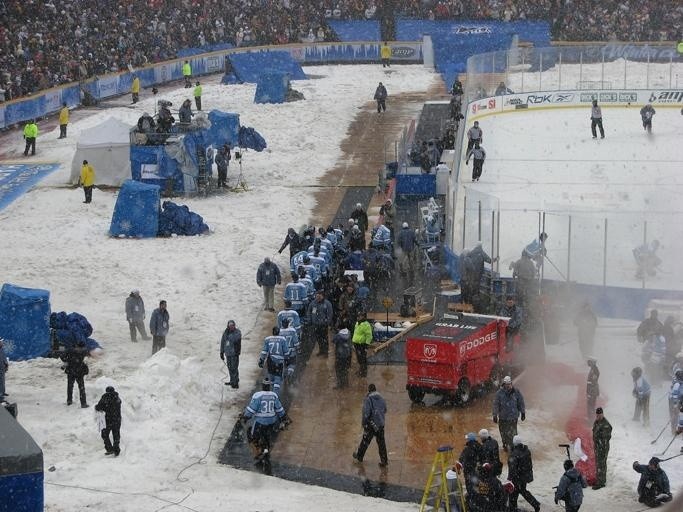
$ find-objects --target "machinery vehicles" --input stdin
[401,311,519,408]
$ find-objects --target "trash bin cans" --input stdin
[436,166,452,194]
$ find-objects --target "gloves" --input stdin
[521,411,525,421]
[492,415,497,423]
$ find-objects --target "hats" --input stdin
[368,384,376,392]
[464,432,475,440]
[649,458,659,469]
[348,198,408,230]
[503,375,512,384]
[478,428,489,438]
[512,434,521,445]
[596,407,603,414]
[563,460,574,470]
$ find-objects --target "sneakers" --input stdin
[353,451,362,461]
[379,462,388,467]
[252,452,265,465]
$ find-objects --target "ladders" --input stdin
[420,446,467,512]
